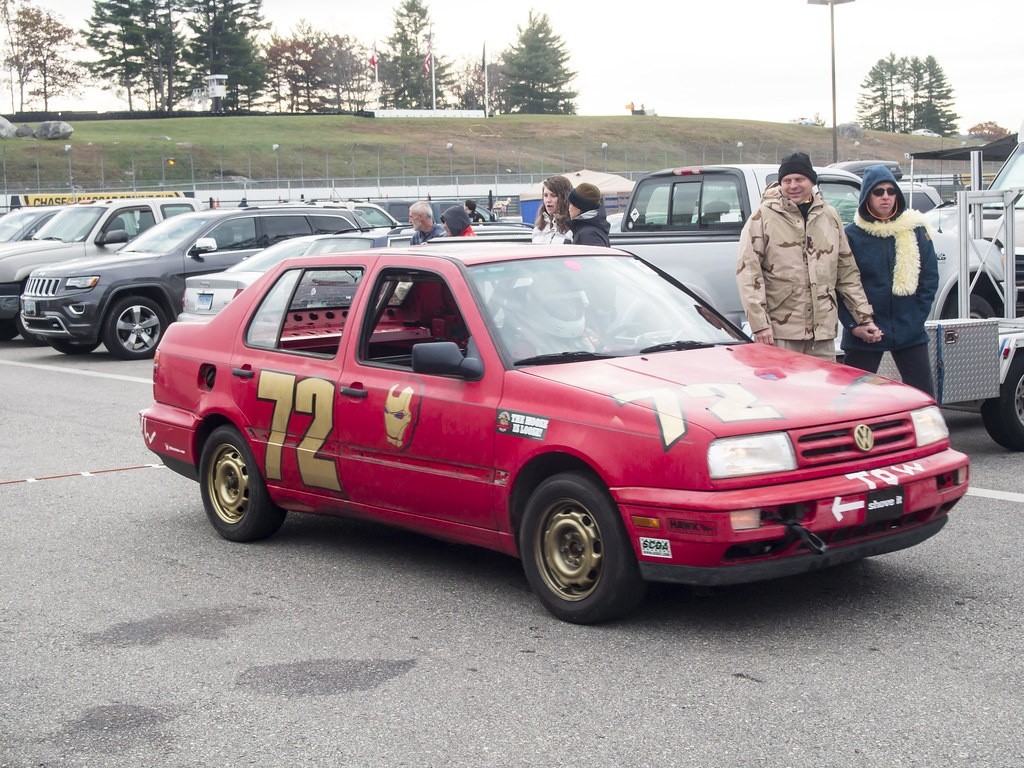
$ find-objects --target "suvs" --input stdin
[19,198,370,362]
[278,198,404,241]
[362,197,501,233]
[302,221,542,301]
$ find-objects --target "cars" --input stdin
[911,128,943,138]
[170,231,332,346]
[137,241,970,628]
[0,206,138,246]
[0,197,198,346]
[604,211,667,238]
[823,139,1024,318]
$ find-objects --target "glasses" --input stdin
[870,187,898,196]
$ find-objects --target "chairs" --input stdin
[696,199,731,223]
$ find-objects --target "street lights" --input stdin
[804,0,859,161]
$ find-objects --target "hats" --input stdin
[568,182,601,212]
[777,152,818,186]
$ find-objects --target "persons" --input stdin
[735,151,881,363]
[565,182,617,337]
[238,198,248,207]
[408,201,448,246]
[463,200,486,222]
[833,164,940,400]
[531,175,573,245]
[440,205,478,238]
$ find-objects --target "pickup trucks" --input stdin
[596,161,1009,371]
